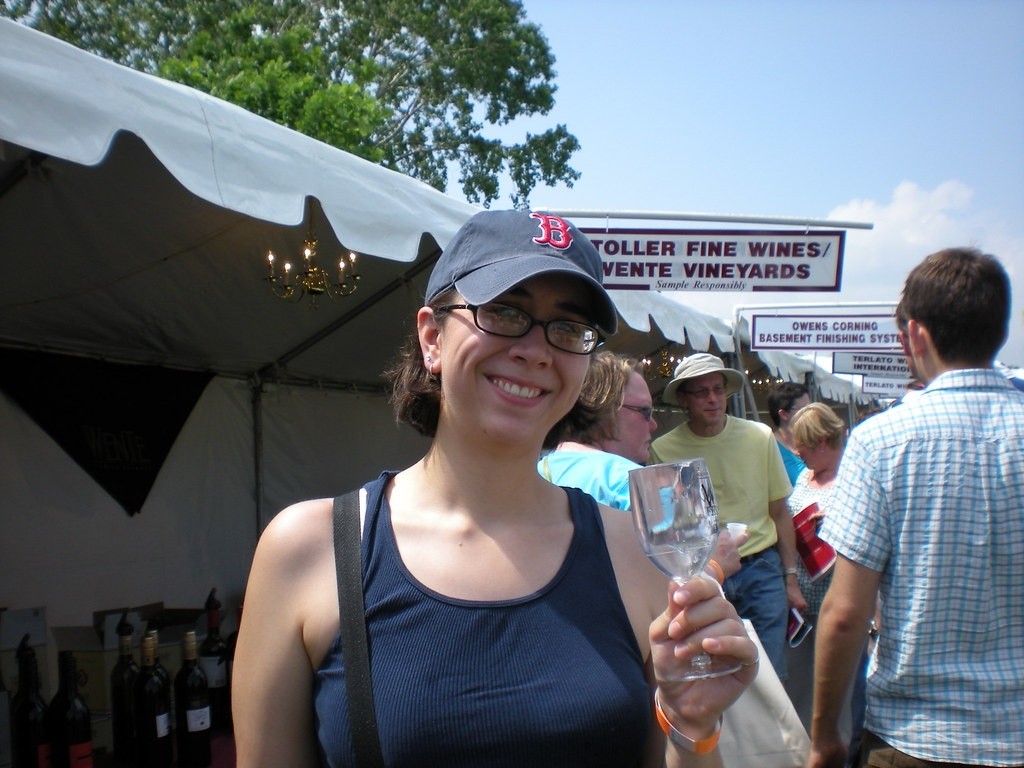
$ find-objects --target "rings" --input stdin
[740,655,759,670]
[818,513,821,518]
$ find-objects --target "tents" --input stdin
[0,14,882,626]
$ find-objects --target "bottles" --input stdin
[109,622,142,768]
[9,648,46,767]
[130,634,171,768]
[43,649,94,768]
[226,606,243,736]
[173,631,212,768]
[145,630,171,709]
[198,600,227,734]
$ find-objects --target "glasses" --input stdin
[676,383,727,399]
[435,302,608,355]
[618,403,652,422]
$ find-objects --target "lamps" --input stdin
[742,368,784,396]
[635,347,688,383]
[266,195,363,310]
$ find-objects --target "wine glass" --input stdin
[627,457,743,682]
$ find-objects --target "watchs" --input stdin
[869,619,879,643]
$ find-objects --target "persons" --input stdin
[231,211,757,768]
[538,353,870,685]
[806,250,1024,768]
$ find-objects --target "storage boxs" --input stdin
[0,601,238,768]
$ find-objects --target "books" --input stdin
[786,608,812,648]
[792,501,838,585]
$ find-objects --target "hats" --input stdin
[663,352,744,407]
[426,209,618,337]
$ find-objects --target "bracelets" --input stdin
[654,686,724,754]
[707,558,725,587]
[784,566,802,577]
